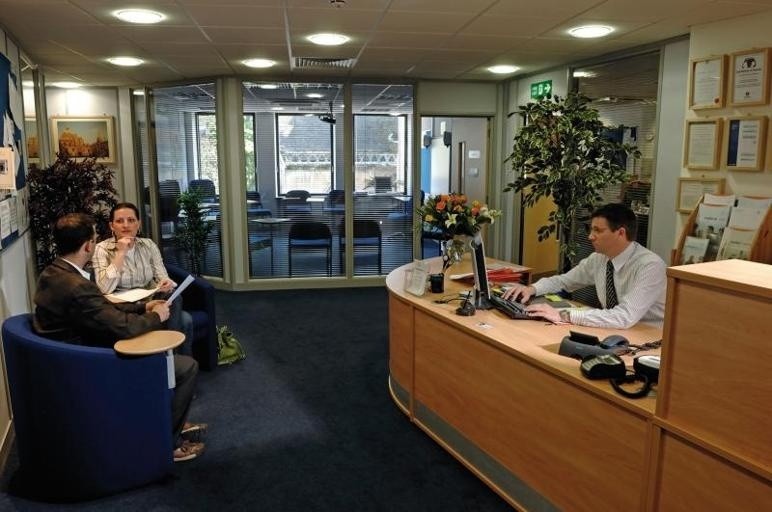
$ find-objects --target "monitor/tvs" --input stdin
[460,234,490,311]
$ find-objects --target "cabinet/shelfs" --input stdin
[670,192,772,266]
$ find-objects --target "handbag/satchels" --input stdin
[217,332,246,366]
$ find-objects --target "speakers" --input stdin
[443,131,452,146]
[423,133,430,146]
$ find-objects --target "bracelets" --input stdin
[559,309,570,324]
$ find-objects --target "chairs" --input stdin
[388,187,445,260]
[320,189,354,229]
[145,178,220,276]
[3,313,175,501]
[246,190,273,239]
[215,215,276,277]
[283,190,313,221]
[338,219,383,275]
[286,221,332,276]
[85,263,217,372]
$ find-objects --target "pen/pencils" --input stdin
[463,291,470,309]
[110,242,118,243]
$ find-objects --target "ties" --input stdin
[605,259,618,309]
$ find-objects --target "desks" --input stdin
[384,252,772,512]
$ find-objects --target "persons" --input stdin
[719,227,724,237]
[91,203,203,401]
[691,223,698,236]
[539,85,542,92]
[706,226,718,235]
[685,255,696,265]
[31,213,207,462]
[502,202,667,331]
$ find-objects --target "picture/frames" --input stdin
[50,116,116,165]
[688,55,727,110]
[721,116,767,172]
[727,48,770,106]
[683,117,723,170]
[25,117,42,164]
[675,176,724,214]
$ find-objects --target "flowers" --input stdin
[412,193,504,262]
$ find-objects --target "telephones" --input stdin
[558,330,634,361]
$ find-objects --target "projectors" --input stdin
[320,115,337,124]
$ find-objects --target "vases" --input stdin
[442,239,464,271]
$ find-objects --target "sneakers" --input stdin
[173,439,206,462]
[180,422,208,441]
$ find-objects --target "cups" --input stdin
[427,273,443,293]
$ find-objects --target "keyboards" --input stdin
[491,292,540,320]
